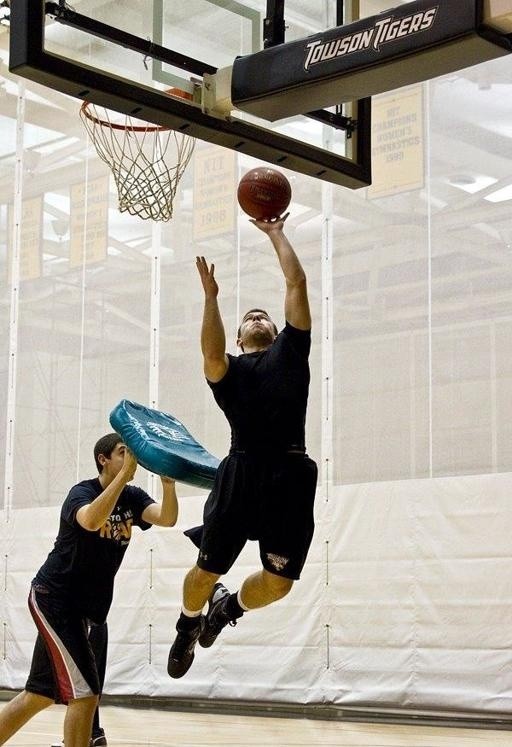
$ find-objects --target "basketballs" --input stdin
[237,167,291,220]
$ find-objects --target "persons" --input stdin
[0,430,179,747]
[51,621,108,747]
[163,211,319,680]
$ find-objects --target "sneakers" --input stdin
[167,610,207,677]
[198,578,230,644]
[51,726,108,747]
